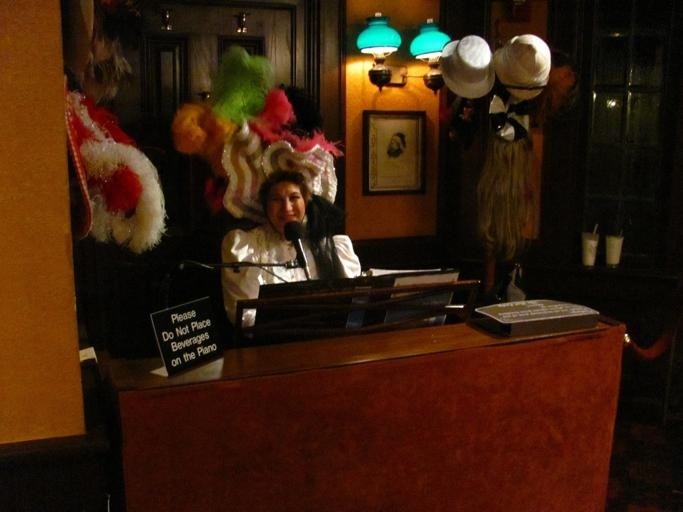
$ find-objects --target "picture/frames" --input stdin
[362,109,427,196]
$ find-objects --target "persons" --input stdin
[218,167,368,347]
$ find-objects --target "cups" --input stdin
[605,235,624,267]
[579,232,600,267]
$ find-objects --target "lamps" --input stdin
[235,10,248,34]
[356,16,407,92]
[159,7,174,32]
[410,21,451,96]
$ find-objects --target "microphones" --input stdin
[284,220,312,281]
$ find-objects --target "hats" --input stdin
[495,34,553,100]
[437,35,495,101]
